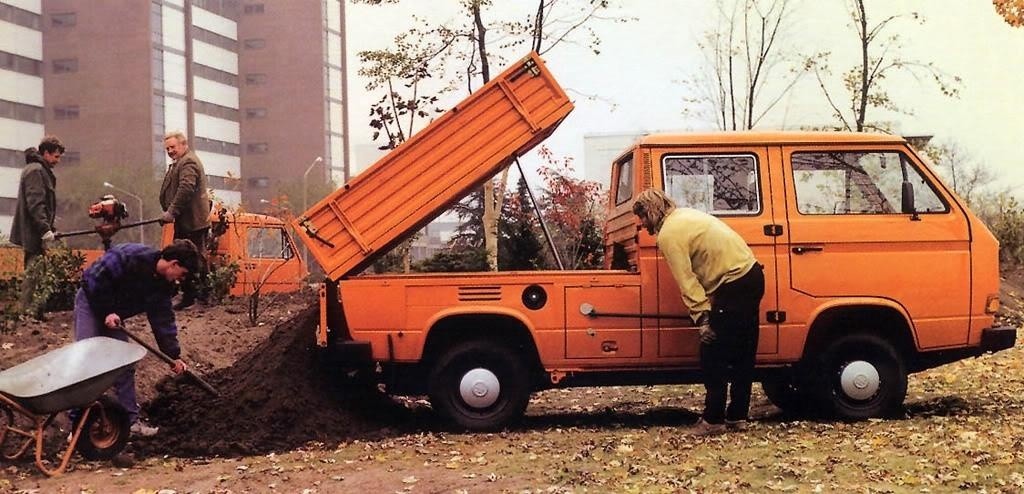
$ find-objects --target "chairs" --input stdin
[748,182,759,213]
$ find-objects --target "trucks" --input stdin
[0,207,310,303]
[293,54,1018,433]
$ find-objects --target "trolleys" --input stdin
[0,337,150,480]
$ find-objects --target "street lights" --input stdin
[302,156,322,274]
[260,197,277,208]
[104,180,144,245]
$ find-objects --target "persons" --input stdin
[9,134,66,322]
[65,238,200,442]
[158,131,211,310]
[632,187,765,434]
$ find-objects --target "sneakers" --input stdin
[129,418,160,440]
[682,416,749,436]
